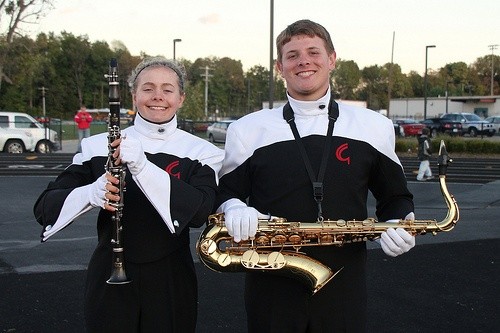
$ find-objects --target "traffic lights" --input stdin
[38,87,49,99]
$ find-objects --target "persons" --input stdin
[74,105,93,154]
[416,128,435,181]
[213,20,415,333]
[32,58,225,333]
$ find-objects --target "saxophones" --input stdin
[194,137,461,295]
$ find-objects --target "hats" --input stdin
[422,127,430,134]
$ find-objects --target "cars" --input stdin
[396,119,426,137]
[485,117,500,135]
[420,118,463,138]
[0,127,33,153]
[207,120,233,143]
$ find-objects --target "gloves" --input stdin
[225,204,276,242]
[380,211,416,256]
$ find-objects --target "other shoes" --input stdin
[418,177,426,181]
[427,175,434,179]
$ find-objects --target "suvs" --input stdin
[439,112,494,137]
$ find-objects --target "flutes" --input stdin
[103,56,135,286]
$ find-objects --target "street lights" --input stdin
[424,45,436,119]
[174,39,182,60]
[446,81,459,112]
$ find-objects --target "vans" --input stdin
[0,112,59,154]
